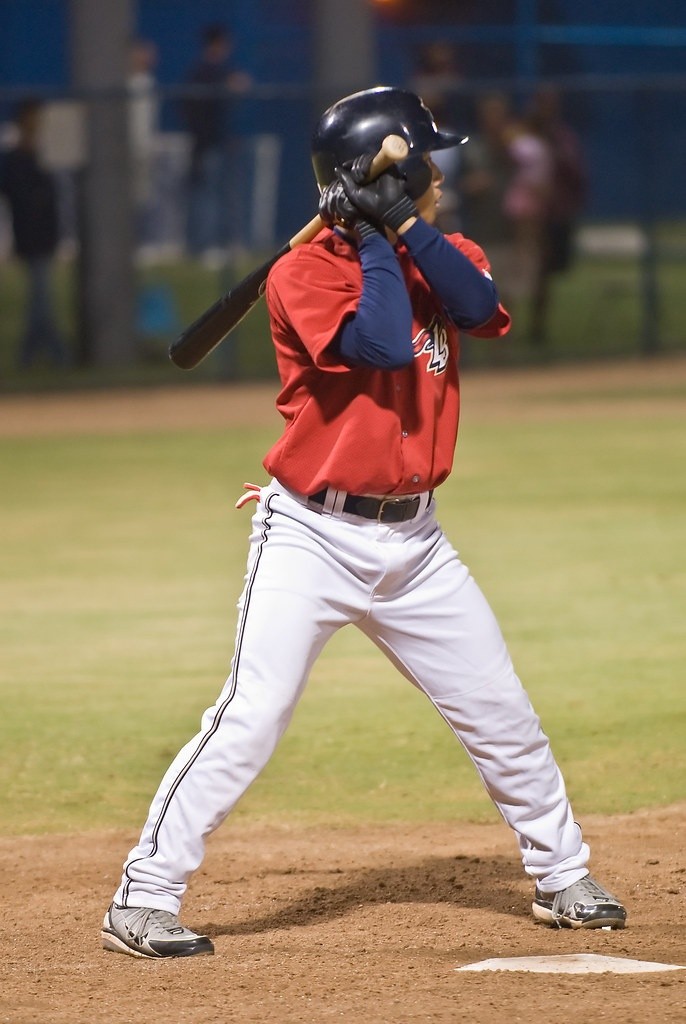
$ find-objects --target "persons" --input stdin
[96,85,626,958]
[0,22,592,374]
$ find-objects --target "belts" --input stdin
[308,486,434,523]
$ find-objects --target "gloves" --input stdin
[334,154,417,232]
[319,180,384,244]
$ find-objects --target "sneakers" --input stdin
[533,877,627,929]
[101,901,216,959]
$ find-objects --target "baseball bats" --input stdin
[167,134,408,373]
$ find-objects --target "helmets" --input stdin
[311,86,467,195]
